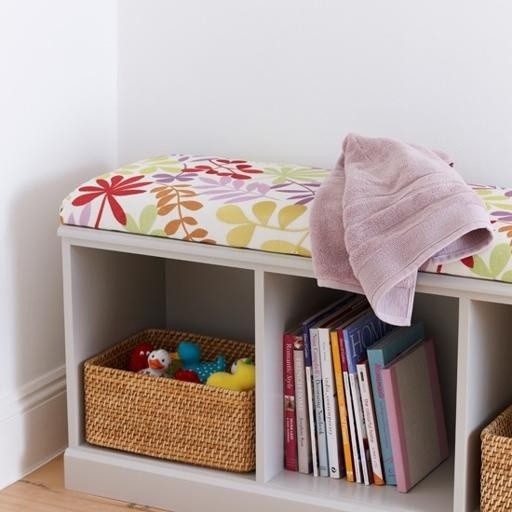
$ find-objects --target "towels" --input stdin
[308,135,494,329]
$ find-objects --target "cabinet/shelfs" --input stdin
[56,225,511,512]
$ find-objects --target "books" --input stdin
[283,293,450,493]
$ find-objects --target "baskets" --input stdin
[82,328,256,473]
[480,405,512,512]
